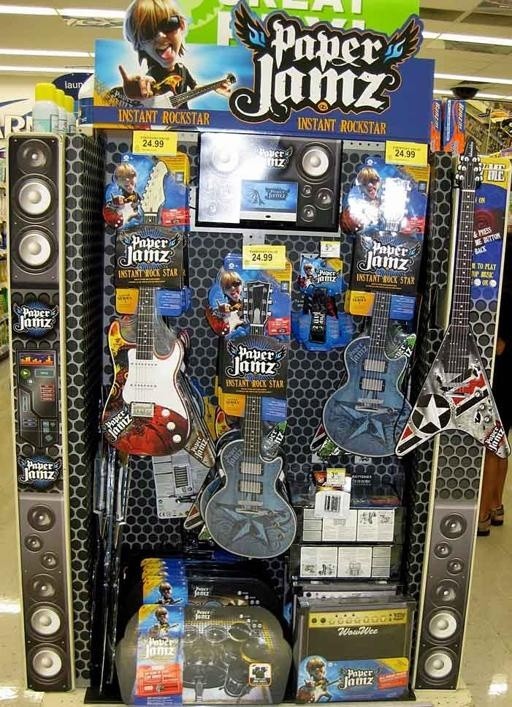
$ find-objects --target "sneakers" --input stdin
[478,506,504,536]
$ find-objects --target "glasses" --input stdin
[225,281,243,288]
[119,172,135,181]
[311,664,324,670]
[139,16,181,39]
[364,176,378,185]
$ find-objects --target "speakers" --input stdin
[199,132,340,231]
[19,494,72,695]
[10,133,60,285]
[411,504,480,690]
